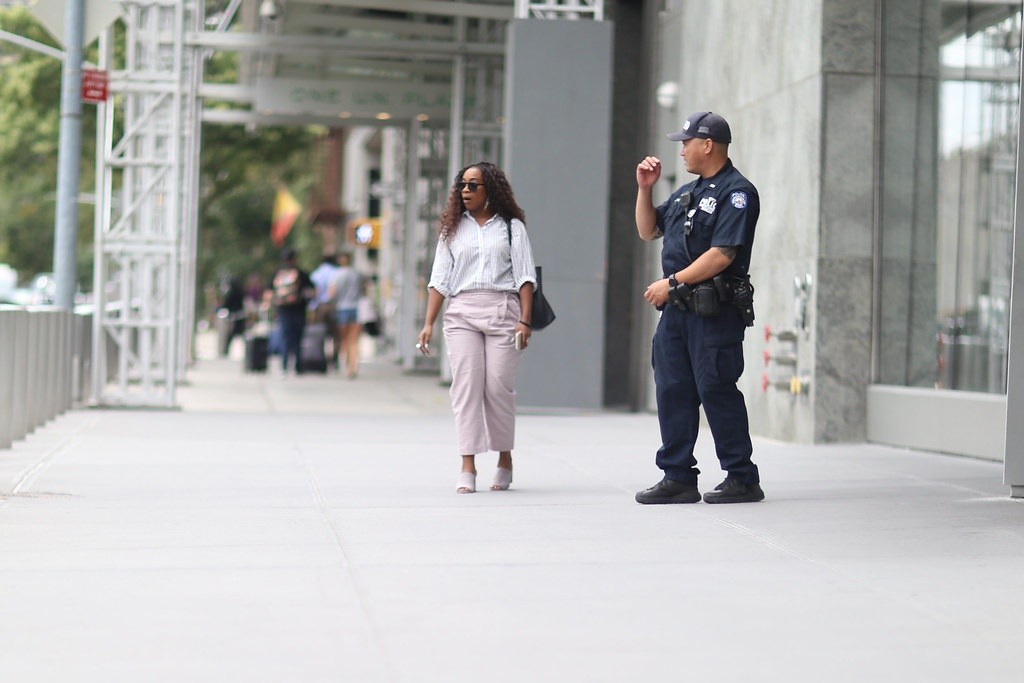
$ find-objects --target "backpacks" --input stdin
[272,268,300,306]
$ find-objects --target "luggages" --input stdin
[296,317,326,373]
[245,337,268,371]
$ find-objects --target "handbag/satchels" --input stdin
[508,218,556,330]
[355,298,378,324]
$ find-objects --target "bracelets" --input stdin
[518,320,531,328]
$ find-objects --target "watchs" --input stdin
[668,274,678,287]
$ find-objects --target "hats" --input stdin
[667,112,731,143]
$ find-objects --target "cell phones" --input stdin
[515,330,523,350]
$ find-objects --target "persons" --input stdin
[632,107,765,506]
[269,250,315,372]
[415,157,540,496]
[309,243,363,378]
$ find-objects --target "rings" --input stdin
[416,344,421,348]
[526,339,529,343]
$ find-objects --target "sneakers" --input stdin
[703,475,764,504]
[636,475,702,504]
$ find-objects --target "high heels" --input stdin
[456,468,476,493]
[489,464,512,490]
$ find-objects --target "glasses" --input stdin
[456,181,486,192]
